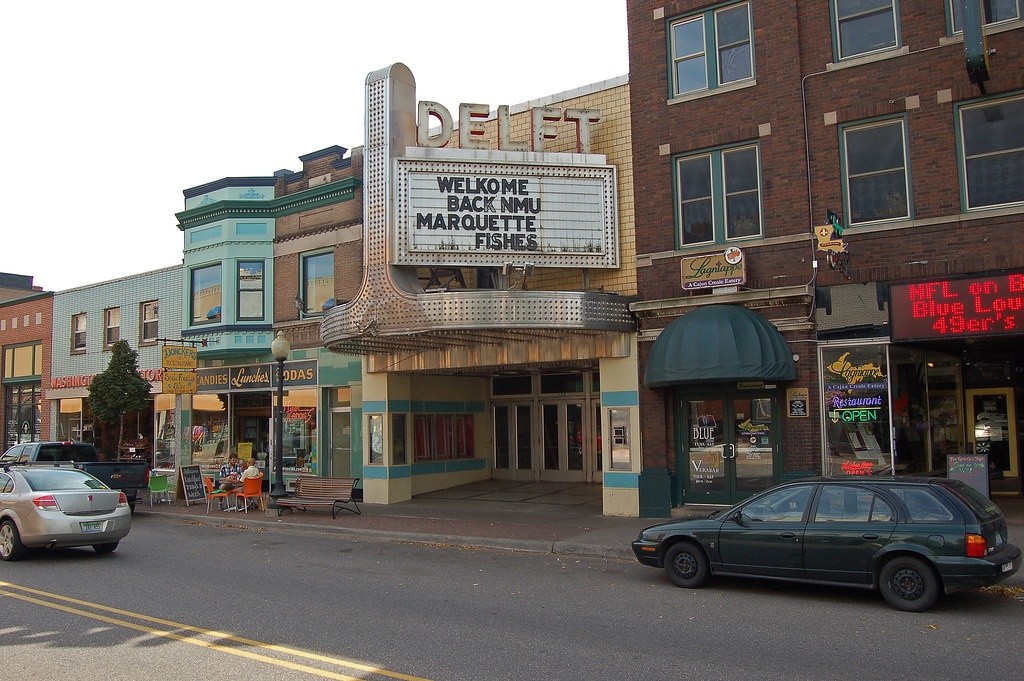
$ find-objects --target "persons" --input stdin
[227,458,259,509]
[218,454,248,511]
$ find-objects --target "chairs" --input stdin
[235,471,265,514]
[203,476,234,515]
[149,474,176,508]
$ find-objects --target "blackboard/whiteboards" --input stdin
[945,454,991,500]
[180,464,207,502]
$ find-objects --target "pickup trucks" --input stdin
[0,441,149,512]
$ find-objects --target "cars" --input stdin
[266,436,312,466]
[827,419,891,467]
[689,429,772,481]
[0,464,132,562]
[577,424,601,456]
[630,473,1023,613]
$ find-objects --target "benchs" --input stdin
[275,477,361,520]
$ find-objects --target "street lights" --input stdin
[268,330,290,509]
